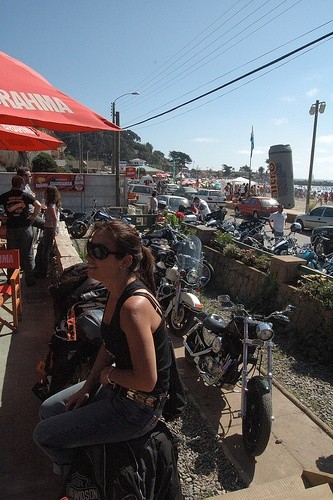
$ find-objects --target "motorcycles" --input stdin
[69,192,132,239]
[132,224,296,457]
[194,206,333,277]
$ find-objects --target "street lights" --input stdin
[110,92,140,174]
[305,100,327,212]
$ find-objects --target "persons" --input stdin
[147,190,158,214]
[223,182,260,196]
[33,220,170,469]
[195,180,202,190]
[157,181,168,195]
[269,204,286,242]
[0,176,42,286]
[16,166,44,270]
[32,186,62,277]
[191,196,211,215]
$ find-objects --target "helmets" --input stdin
[77,310,105,349]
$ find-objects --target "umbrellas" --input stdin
[0,124,64,151]
[0,51,123,132]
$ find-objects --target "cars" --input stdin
[234,196,284,220]
[310,226,333,256]
[127,182,227,223]
[294,205,333,230]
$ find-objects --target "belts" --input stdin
[120,389,154,407]
[122,388,168,408]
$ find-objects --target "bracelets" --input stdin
[107,367,120,385]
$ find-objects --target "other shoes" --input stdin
[34,271,47,278]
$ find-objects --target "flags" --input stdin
[250,126,254,158]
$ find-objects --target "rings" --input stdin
[66,403,72,407]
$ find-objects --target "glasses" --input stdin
[87,241,118,260]
[28,175,32,178]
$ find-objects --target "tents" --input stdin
[141,173,171,184]
[226,177,257,193]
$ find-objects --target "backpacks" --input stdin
[32,263,109,402]
[100,419,184,500]
